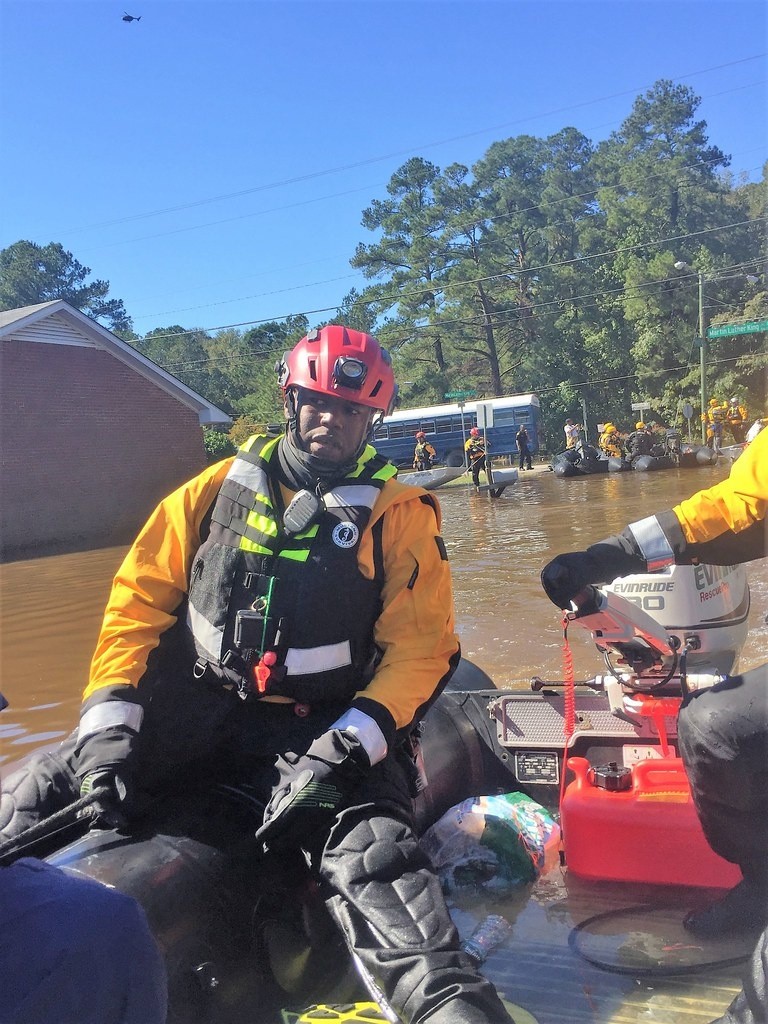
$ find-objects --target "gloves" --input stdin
[540,509,687,612]
[252,696,398,847]
[73,686,146,831]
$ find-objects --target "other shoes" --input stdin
[520,468,525,470]
[528,467,535,470]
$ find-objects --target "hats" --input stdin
[710,399,719,405]
[636,422,645,430]
[607,426,617,434]
[604,423,613,431]
[729,398,739,404]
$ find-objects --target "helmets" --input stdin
[415,431,426,439]
[470,427,480,438]
[274,323,401,416]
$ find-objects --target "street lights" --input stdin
[578,397,588,442]
[674,261,708,446]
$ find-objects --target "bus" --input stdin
[369,394,539,468]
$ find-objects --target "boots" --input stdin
[713,436,725,456]
[680,791,768,937]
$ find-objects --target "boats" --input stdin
[550,439,750,477]
[397,467,467,490]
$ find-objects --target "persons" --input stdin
[541,426,768,1024]
[465,428,491,487]
[0,323,513,1024]
[413,432,436,470]
[601,422,626,459]
[563,419,583,449]
[626,422,660,461]
[516,425,534,470]
[701,398,747,450]
